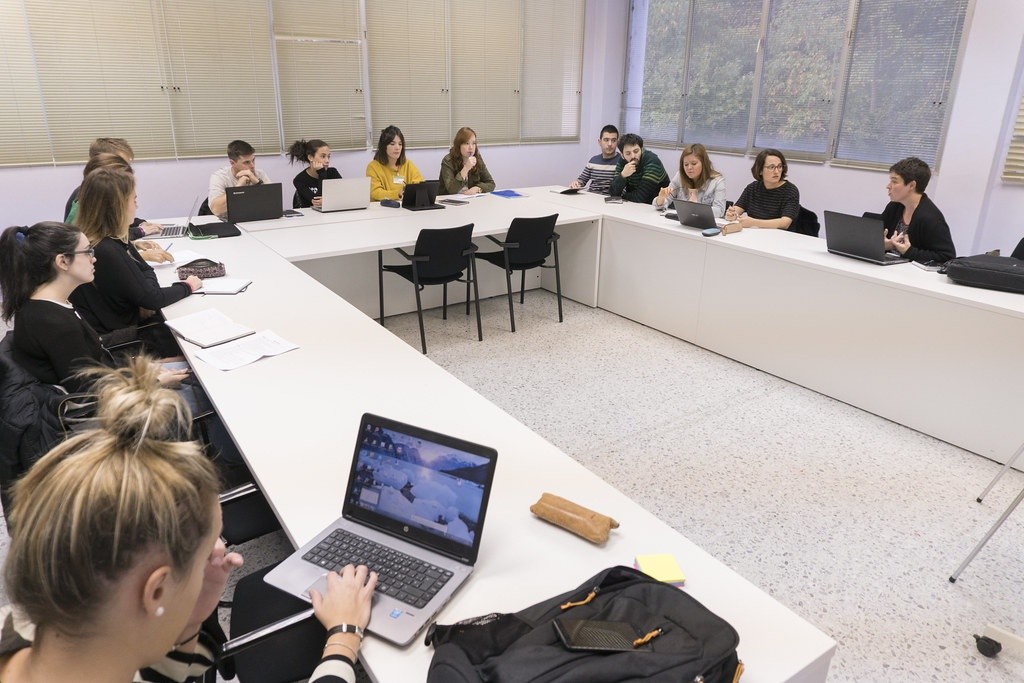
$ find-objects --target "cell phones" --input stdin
[552,618,653,653]
[161,361,193,373]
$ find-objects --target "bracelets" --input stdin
[322,642,358,662]
[478,187,482,193]
[325,623,363,642]
[174,633,199,649]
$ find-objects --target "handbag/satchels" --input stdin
[937,254,1024,293]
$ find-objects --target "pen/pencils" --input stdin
[165,243,172,251]
[470,152,471,156]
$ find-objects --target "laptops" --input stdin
[218,183,283,224]
[142,197,198,240]
[263,414,498,648]
[824,211,910,265]
[310,177,371,213]
[672,199,727,229]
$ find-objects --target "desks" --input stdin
[134,186,1024,682]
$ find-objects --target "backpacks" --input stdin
[424,565,744,683]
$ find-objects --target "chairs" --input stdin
[379,223,482,354]
[293,190,304,209]
[797,205,820,238]
[1,322,329,683]
[198,196,214,217]
[466,213,564,332]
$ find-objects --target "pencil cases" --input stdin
[720,223,742,236]
[177,258,226,280]
[530,492,620,543]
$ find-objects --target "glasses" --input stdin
[763,163,785,172]
[55,247,94,258]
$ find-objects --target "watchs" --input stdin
[255,178,263,185]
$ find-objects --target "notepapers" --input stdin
[634,554,686,588]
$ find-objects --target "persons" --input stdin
[208,140,272,218]
[437,127,496,196]
[64,137,202,356]
[653,144,727,219]
[725,148,800,231]
[0,222,246,474]
[366,125,425,202]
[570,125,626,196]
[880,158,956,265]
[609,133,674,210]
[285,139,343,208]
[0,351,378,683]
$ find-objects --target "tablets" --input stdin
[402,182,439,208]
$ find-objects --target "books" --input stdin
[660,209,677,216]
[913,259,941,271]
[164,307,255,349]
[439,199,469,206]
[282,209,303,217]
[604,197,623,204]
[551,187,585,194]
[191,277,253,295]
